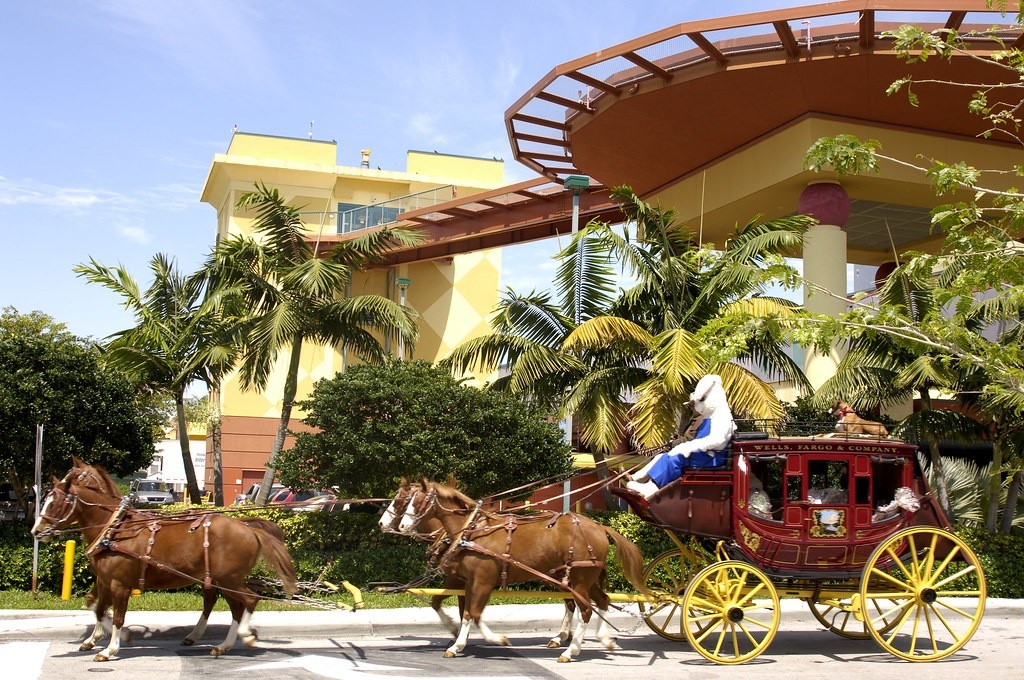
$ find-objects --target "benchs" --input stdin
[682,443,733,531]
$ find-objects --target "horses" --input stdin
[30,451,297,662]
[377,472,655,664]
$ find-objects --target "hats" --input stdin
[682,391,697,405]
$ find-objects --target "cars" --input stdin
[234,480,364,514]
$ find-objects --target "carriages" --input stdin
[31,417,988,666]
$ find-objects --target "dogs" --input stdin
[827,399,888,439]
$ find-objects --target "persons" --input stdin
[619,374,738,501]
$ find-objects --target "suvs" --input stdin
[128,476,175,510]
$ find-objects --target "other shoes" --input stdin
[619,466,633,483]
[626,479,660,501]
